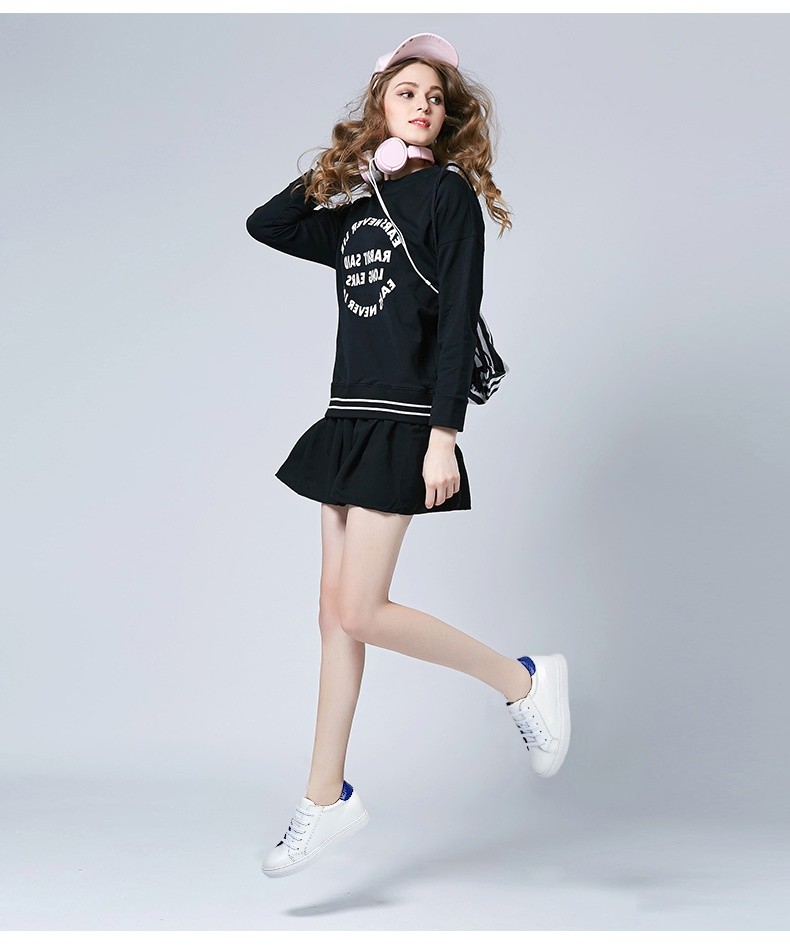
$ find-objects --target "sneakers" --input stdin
[262,782,369,879]
[372,33,458,90]
[507,653,572,778]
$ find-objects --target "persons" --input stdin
[239,54,578,880]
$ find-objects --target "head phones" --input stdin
[357,137,434,184]
